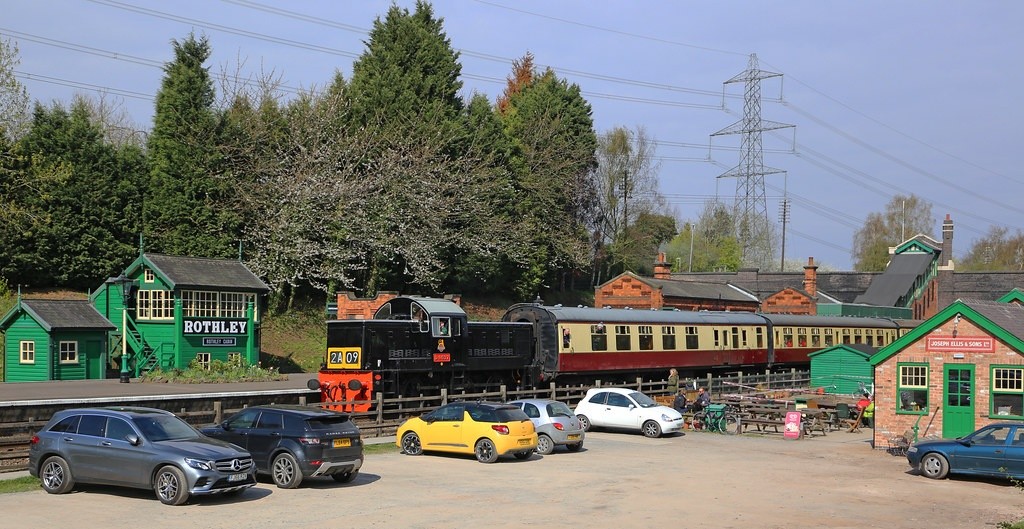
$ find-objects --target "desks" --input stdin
[743,407,828,438]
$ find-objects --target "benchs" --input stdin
[679,397,841,438]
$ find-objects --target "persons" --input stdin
[563,328,570,345]
[593,321,604,349]
[783,336,821,348]
[439,319,448,336]
[667,368,679,396]
[673,388,689,411]
[690,386,711,411]
[849,393,875,428]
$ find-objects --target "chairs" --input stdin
[984,435,996,444]
[448,409,459,421]
[844,406,866,434]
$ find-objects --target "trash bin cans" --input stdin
[704,403,727,431]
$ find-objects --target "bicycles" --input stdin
[691,398,739,435]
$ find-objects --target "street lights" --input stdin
[114,269,135,383]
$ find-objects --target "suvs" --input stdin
[197,403,364,489]
[28,406,258,506]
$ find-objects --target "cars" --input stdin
[396,401,539,463]
[906,421,1024,481]
[504,399,585,455]
[574,387,685,438]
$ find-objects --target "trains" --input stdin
[307,298,927,412]
